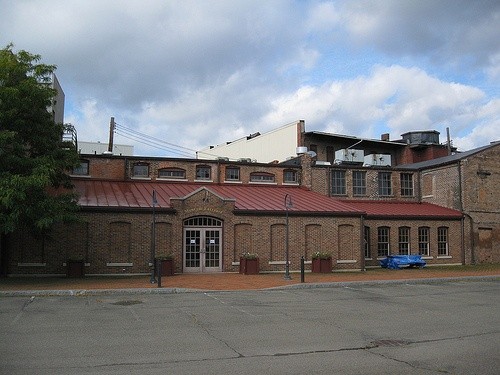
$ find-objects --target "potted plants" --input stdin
[155,254,174,276]
[312,251,332,273]
[239,252,259,275]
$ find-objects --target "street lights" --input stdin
[151,190,158,283]
[282,192,294,280]
